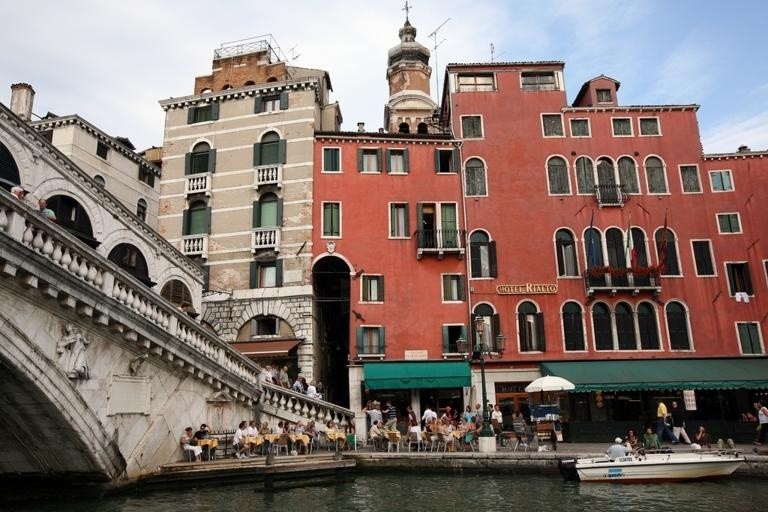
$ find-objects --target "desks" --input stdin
[197,439,218,461]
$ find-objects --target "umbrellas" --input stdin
[524,374,575,405]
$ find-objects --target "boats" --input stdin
[556,447,749,482]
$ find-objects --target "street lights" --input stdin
[454,311,506,454]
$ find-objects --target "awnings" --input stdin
[363,363,473,389]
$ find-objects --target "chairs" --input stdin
[243,430,347,458]
[371,422,537,453]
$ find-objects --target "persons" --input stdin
[177,301,192,315]
[182,424,215,462]
[362,400,505,453]
[266,366,322,400]
[233,420,355,458]
[604,399,768,463]
[513,410,523,443]
